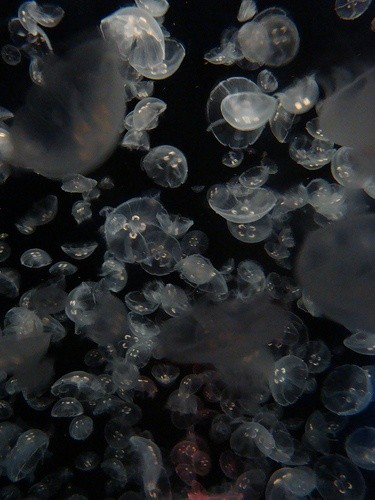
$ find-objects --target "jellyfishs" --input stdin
[0,0,375,500]
